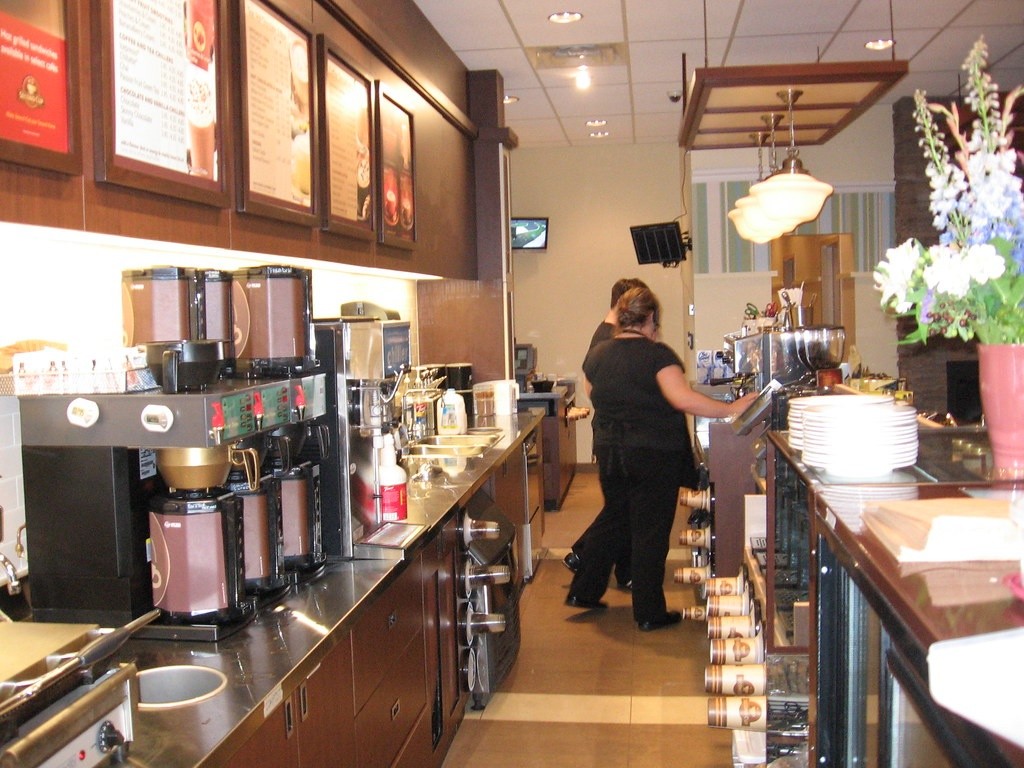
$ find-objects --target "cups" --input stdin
[462,513,510,636]
[568,405,591,422]
[385,190,411,223]
[674,487,766,733]
[419,363,472,390]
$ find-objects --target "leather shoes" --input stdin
[566,592,607,610]
[639,611,681,631]
[563,552,581,575]
[616,574,633,592]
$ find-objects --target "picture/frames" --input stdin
[2,1,421,248]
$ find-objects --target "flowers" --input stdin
[870,36,1024,344]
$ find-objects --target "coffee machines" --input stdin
[16,264,327,642]
[783,326,844,393]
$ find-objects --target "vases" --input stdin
[977,342,1024,481]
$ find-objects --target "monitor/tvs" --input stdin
[510,217,548,249]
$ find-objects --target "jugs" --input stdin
[493,379,520,416]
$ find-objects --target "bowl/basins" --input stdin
[530,380,555,393]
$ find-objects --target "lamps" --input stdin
[728,90,832,243]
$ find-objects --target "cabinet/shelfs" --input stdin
[207,519,471,768]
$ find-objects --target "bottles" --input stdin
[378,433,408,524]
[436,388,467,436]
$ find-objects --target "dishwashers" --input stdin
[522,422,543,580]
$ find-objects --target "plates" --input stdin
[384,201,414,230]
[787,394,919,478]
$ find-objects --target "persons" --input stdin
[562,278,649,590]
[565,286,759,632]
